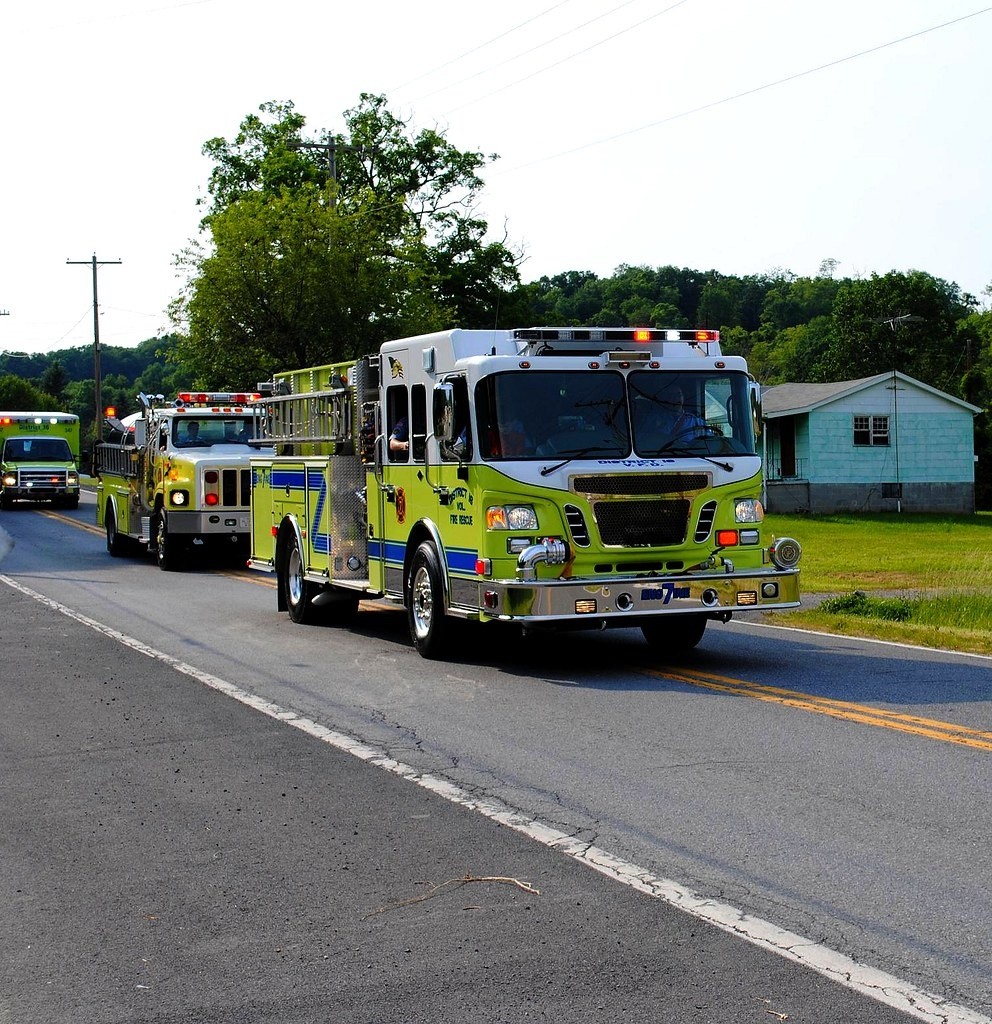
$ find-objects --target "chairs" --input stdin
[615,397,664,438]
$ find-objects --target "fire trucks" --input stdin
[90,389,280,571]
[247,325,804,659]
[0,411,82,509]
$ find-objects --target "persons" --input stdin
[388,415,411,453]
[448,383,539,457]
[239,421,265,443]
[639,381,717,455]
[176,421,206,443]
